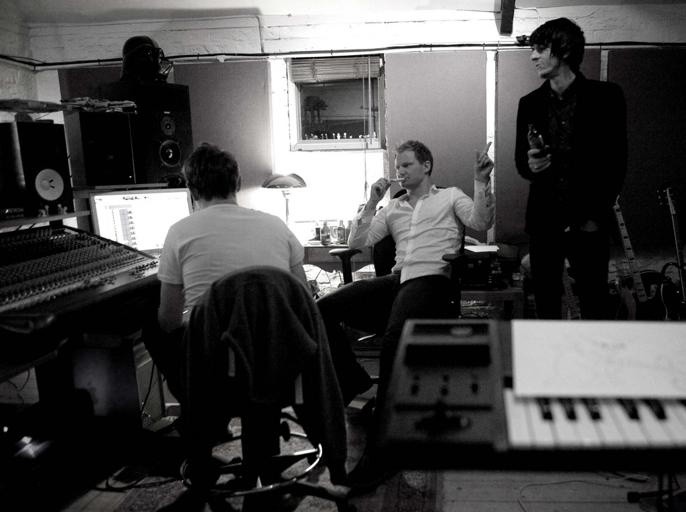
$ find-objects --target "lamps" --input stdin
[262,172,307,227]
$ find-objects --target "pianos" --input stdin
[488,318,686,473]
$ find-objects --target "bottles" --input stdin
[527,122,546,159]
[320,220,353,245]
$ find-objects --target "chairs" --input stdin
[329,186,465,319]
[171,270,323,497]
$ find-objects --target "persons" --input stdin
[514,17,631,318]
[316,140,495,491]
[141,142,308,464]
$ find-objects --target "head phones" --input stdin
[557,30,584,64]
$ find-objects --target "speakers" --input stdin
[0,83,194,220]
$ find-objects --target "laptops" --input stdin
[88,188,193,258]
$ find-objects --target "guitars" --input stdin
[612,187,685,321]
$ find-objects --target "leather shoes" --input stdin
[344,366,372,408]
